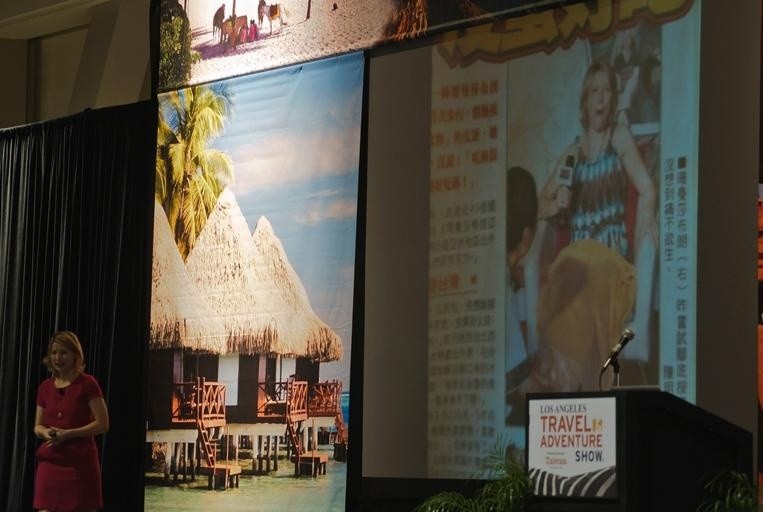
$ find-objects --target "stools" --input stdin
[264,400,286,415]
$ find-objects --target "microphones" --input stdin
[601,329,634,374]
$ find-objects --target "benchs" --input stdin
[290,451,328,478]
[199,463,242,490]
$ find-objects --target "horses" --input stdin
[258,0,287,35]
[213,4,256,51]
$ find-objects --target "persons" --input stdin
[33,330,109,511]
[540,62,659,262]
[507,166,537,373]
